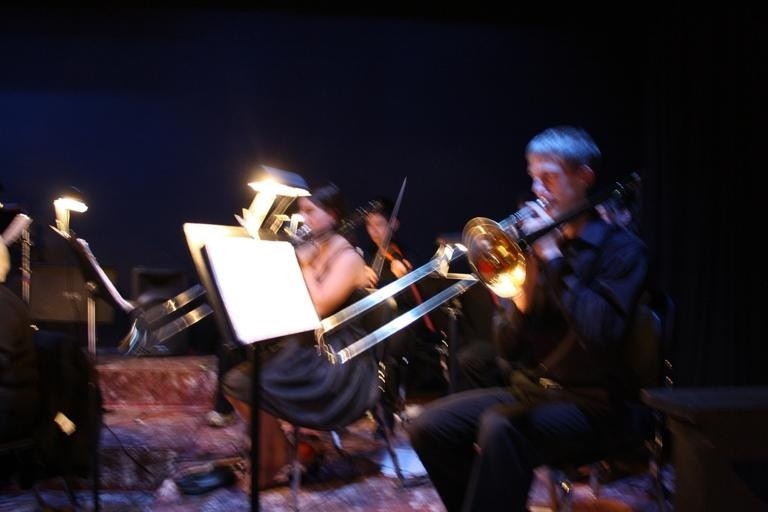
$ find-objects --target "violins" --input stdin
[372,174,434,334]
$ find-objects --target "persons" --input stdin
[220,180,380,488]
[1,234,46,445]
[359,200,423,307]
[405,127,656,511]
[207,338,244,426]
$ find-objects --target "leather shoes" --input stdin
[223,452,250,472]
[231,460,310,494]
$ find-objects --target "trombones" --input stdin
[132,199,381,357]
[314,171,644,367]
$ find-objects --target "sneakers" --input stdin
[205,410,233,426]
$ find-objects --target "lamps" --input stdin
[247,165,311,198]
[51,186,88,213]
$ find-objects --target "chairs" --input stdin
[292,291,405,511]
[535,305,674,512]
